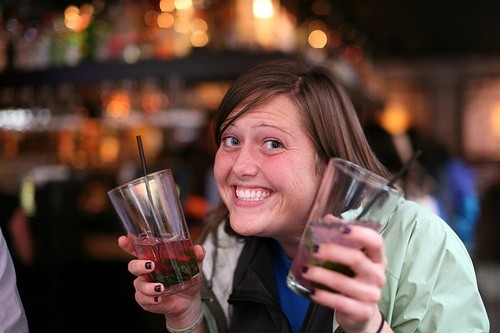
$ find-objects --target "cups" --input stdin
[108,168,203,297]
[286,157,402,300]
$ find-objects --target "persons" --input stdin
[0,111,220,333]
[118,60,490,333]
[362,97,500,259]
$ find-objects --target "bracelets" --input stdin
[377,310,384,333]
[165,310,202,333]
